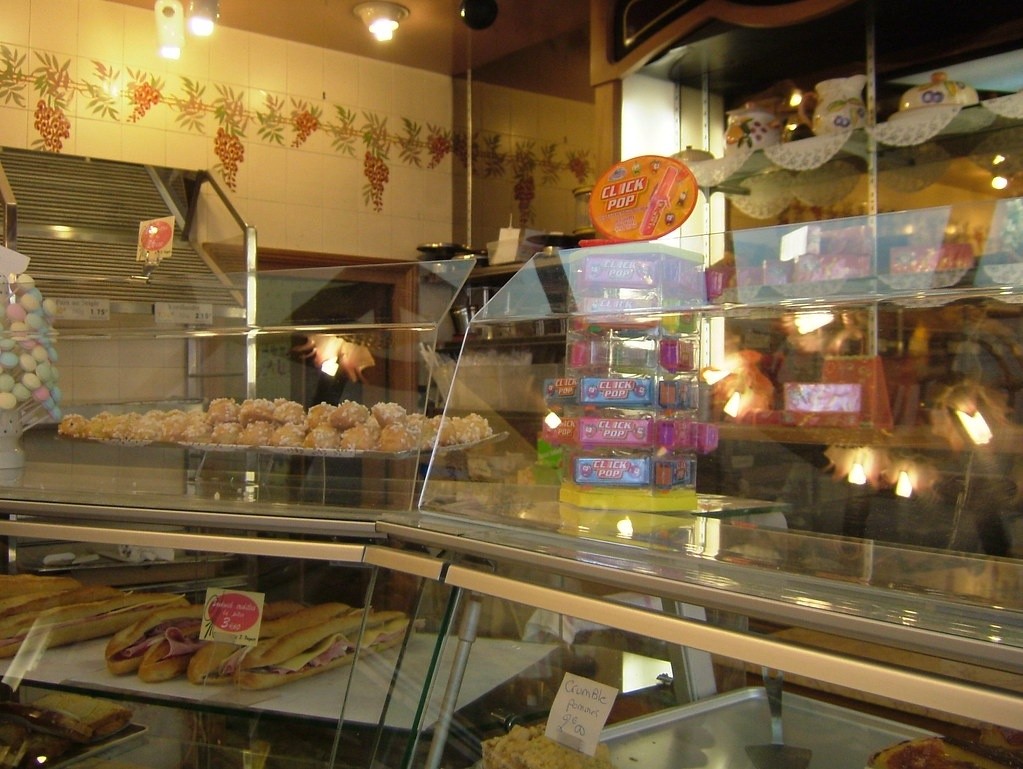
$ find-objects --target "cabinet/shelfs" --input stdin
[422,242,703,428]
[0,258,565,769]
[335,88,1023,768]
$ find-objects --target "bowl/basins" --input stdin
[451,287,568,336]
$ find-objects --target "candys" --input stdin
[0,275,62,421]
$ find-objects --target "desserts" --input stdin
[874,737,1013,769]
[480,723,619,769]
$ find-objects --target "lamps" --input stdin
[987,153,1013,191]
[186,1,220,37]
[352,2,409,42]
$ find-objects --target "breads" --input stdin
[0,571,416,690]
[0,693,133,769]
[59,397,491,452]
